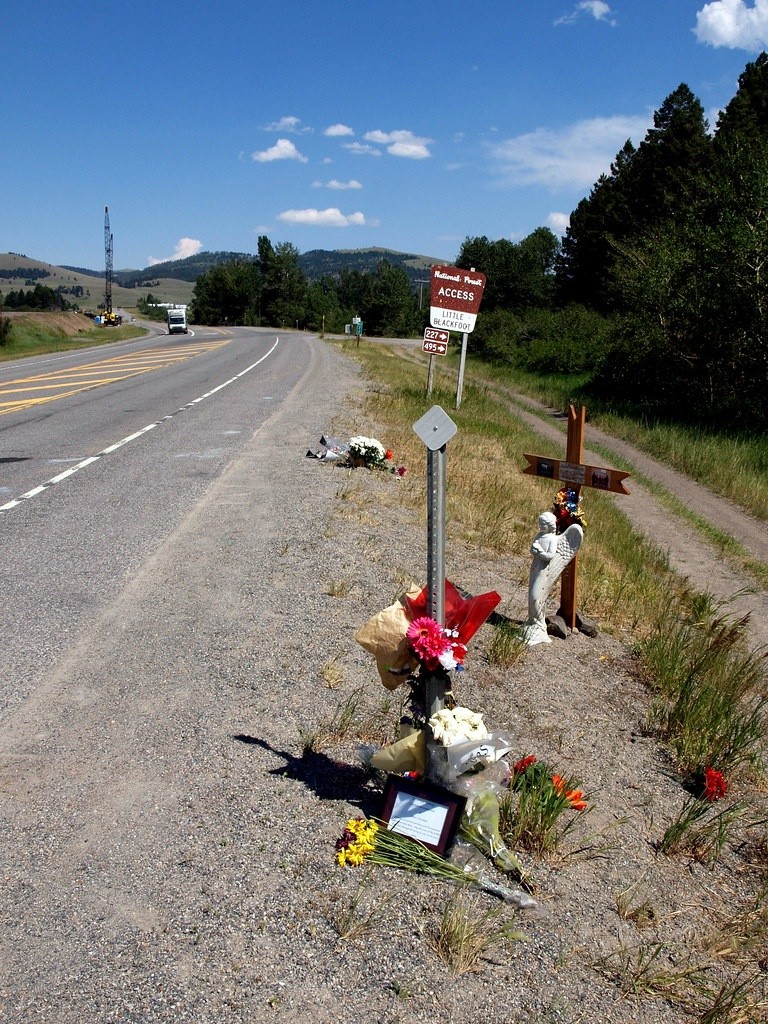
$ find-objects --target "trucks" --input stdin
[168,309,188,334]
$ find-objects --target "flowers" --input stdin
[550,488,589,533]
[321,435,405,475]
[703,768,728,802]
[334,616,587,906]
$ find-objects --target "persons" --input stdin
[519,511,556,636]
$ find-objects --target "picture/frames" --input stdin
[378,773,469,858]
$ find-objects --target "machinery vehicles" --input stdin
[100,206,121,326]
